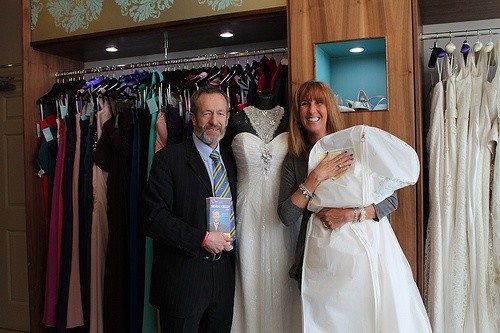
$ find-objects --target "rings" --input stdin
[323,222,329,230]
[338,165,342,168]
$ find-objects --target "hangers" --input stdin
[429,28,494,68]
[38,48,288,104]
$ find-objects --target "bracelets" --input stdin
[298,184,312,199]
[353,207,367,222]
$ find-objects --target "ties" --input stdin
[209,149,237,245]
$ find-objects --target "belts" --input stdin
[204,252,227,261]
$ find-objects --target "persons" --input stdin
[278,79,434,333]
[146,85,238,333]
[210,210,229,233]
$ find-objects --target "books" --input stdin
[206,197,231,248]
